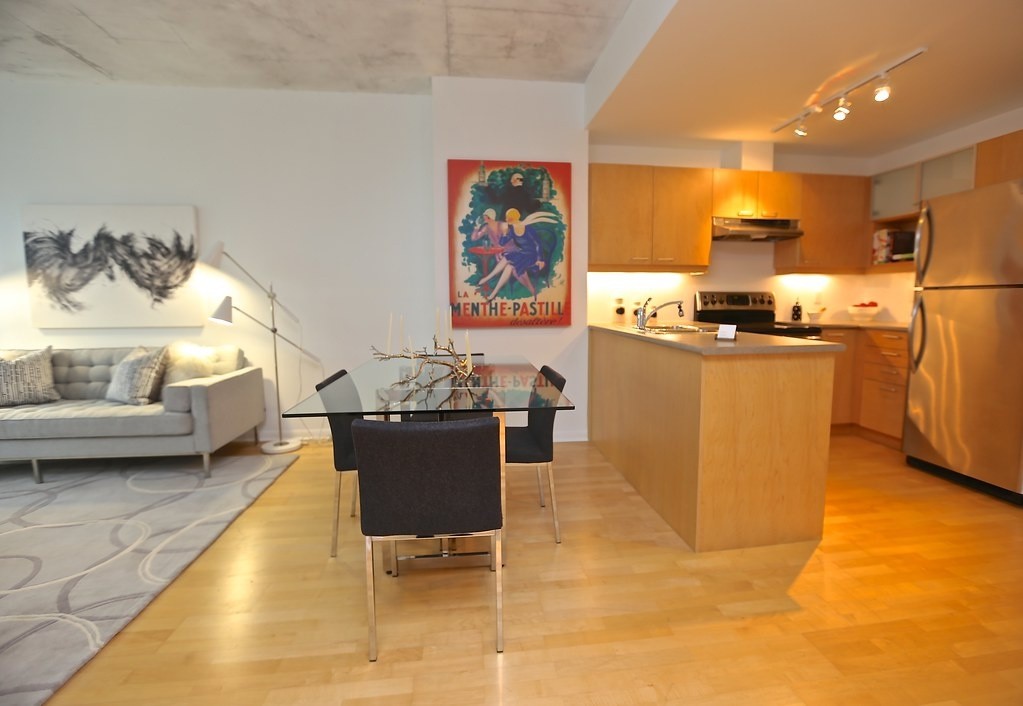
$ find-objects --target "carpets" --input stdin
[0,451,298,706]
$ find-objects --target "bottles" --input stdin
[791,301,802,323]
[648,305,657,325]
[631,301,641,325]
[612,297,625,326]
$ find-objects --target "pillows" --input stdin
[0,349,63,407]
[105,347,170,406]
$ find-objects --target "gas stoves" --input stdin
[693,291,822,337]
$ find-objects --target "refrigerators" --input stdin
[901,178,1023,510]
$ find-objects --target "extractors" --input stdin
[712,217,804,242]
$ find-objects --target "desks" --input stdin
[282,356,576,553]
[589,325,836,556]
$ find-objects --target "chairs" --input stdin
[317,353,567,663]
[0,346,265,479]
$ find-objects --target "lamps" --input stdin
[195,242,321,454]
[795,70,893,138]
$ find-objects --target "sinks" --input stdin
[649,329,718,333]
[633,325,695,330]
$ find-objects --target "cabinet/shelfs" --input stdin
[823,326,912,452]
[587,161,873,271]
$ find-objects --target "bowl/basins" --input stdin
[848,305,882,322]
[807,312,822,324]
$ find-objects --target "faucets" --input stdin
[636,298,684,329]
